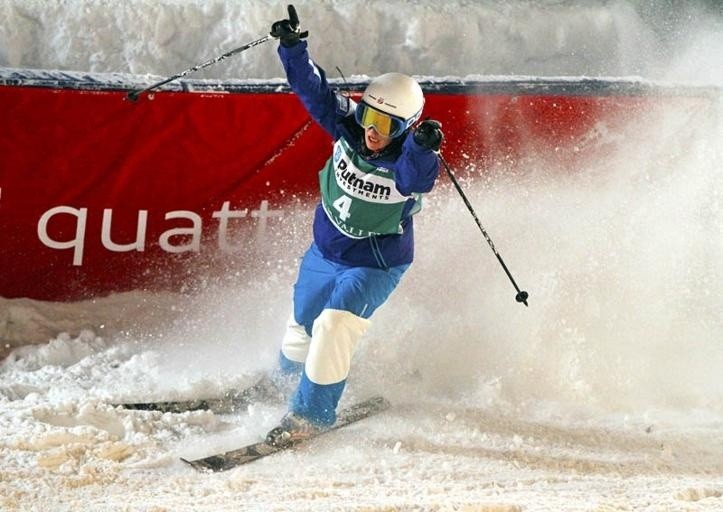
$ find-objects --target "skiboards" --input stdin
[112,388,390,473]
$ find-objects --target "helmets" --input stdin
[360,72,426,133]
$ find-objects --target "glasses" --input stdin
[354,101,405,139]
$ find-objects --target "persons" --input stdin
[229,3,446,449]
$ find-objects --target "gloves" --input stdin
[413,118,446,152]
[269,4,308,49]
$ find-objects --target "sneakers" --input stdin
[264,414,336,449]
[223,375,289,405]
